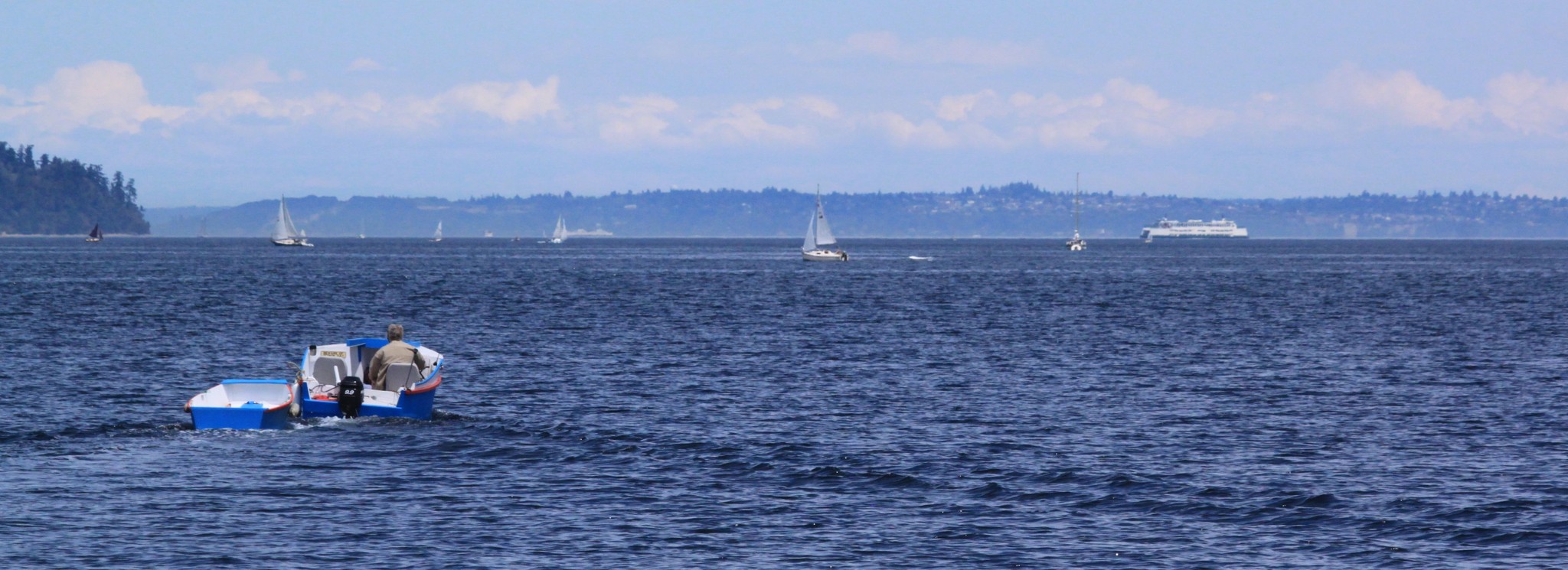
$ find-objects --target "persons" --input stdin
[368,323,426,391]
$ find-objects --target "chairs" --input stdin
[385,363,421,392]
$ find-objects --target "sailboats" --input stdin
[269,195,314,246]
[547,213,567,243]
[800,184,850,262]
[1065,171,1087,251]
[428,220,443,242]
[83,222,104,242]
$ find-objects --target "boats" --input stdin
[909,256,932,261]
[1139,212,1249,238]
[183,378,294,431]
[296,337,445,422]
